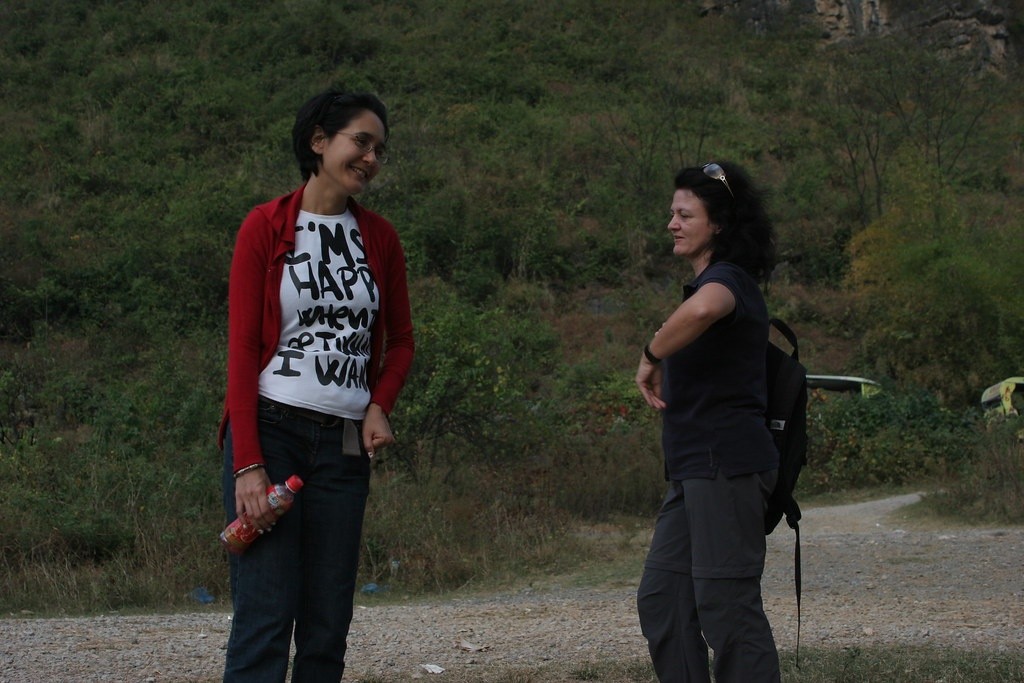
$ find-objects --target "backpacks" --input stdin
[763,317,807,535]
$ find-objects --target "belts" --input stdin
[260,394,360,456]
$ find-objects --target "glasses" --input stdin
[336,130,389,164]
[701,163,736,197]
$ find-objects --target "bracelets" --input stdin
[233,461,268,480]
[644,344,665,367]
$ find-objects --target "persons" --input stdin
[215,91,415,683]
[634,159,781,683]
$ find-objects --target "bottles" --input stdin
[219,475,304,553]
